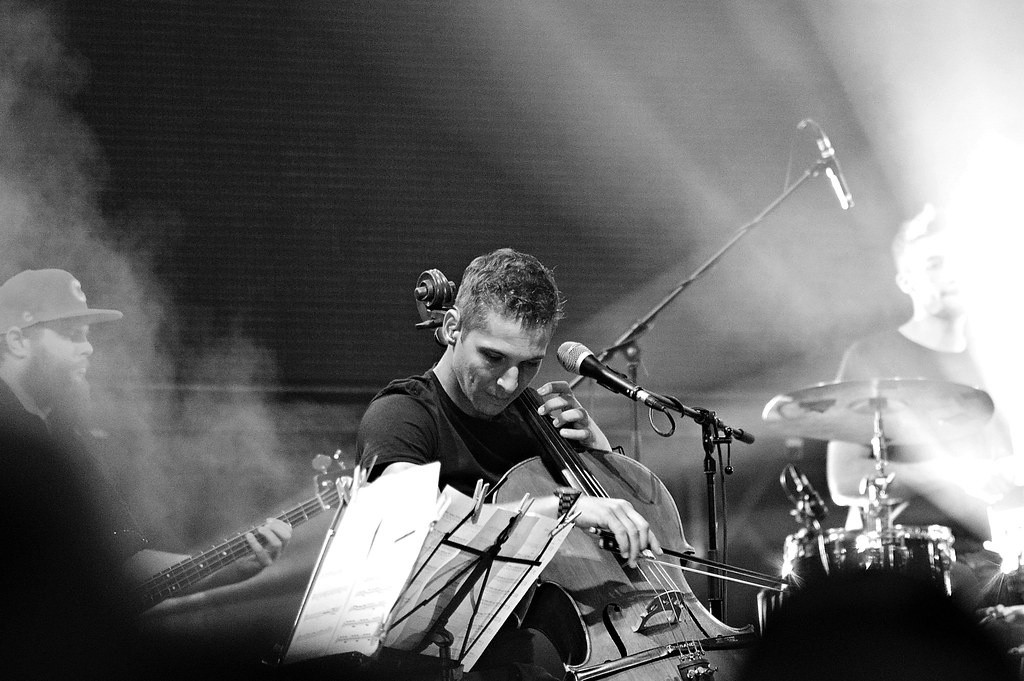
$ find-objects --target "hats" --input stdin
[0,268,124,335]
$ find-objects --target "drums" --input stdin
[771,520,958,606]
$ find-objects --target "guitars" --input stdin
[123,446,357,617]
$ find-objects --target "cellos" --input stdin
[410,266,761,681]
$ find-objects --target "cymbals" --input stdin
[761,379,997,436]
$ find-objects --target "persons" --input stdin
[0,267,293,680]
[721,201,1024,681]
[354,246,662,681]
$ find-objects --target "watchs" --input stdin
[551,488,580,523]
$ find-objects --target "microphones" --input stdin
[809,124,853,210]
[559,340,665,409]
[791,464,830,518]
